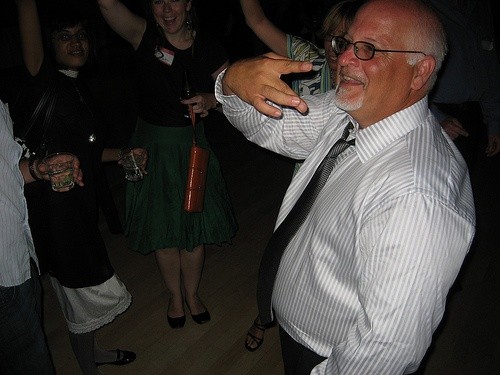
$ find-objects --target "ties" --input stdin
[255,122,355,325]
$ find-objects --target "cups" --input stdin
[43,152,75,192]
[119,145,148,182]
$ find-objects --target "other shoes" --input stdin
[184,296,211,323]
[167,298,185,329]
[96,350,136,366]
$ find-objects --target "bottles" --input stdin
[182,69,199,122]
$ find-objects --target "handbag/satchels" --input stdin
[183,102,209,213]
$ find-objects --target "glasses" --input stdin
[332,35,425,61]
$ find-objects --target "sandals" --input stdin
[245,321,273,352]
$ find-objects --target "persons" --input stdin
[97,0,239,329]
[240,0,365,352]
[19,0,148,375]
[423,0,500,286]
[0,99,85,375]
[215,0,476,375]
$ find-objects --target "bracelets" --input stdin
[28,158,40,180]
[36,160,45,180]
